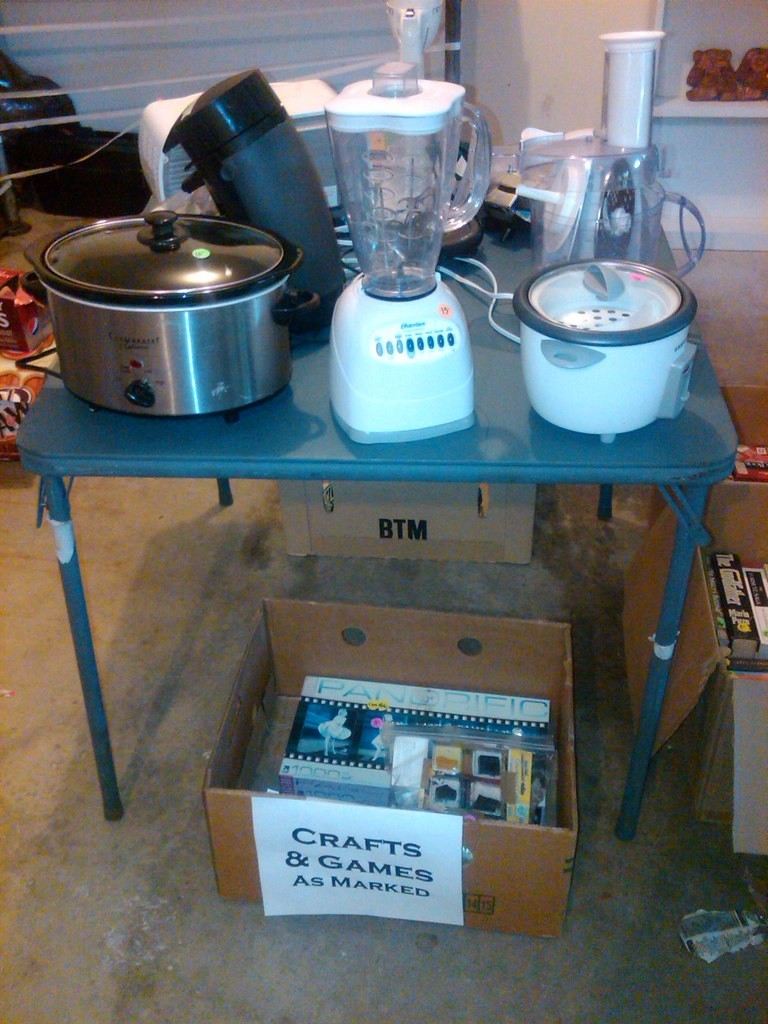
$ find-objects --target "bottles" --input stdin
[163,68,345,333]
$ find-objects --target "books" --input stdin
[733,459,768,483]
[706,551,768,670]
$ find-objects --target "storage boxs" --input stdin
[0,271,58,465]
[202,598,580,939]
[619,248,768,857]
[277,479,537,565]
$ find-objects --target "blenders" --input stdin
[325,61,492,446]
[516,30,707,276]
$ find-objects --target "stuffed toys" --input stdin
[686,46,768,102]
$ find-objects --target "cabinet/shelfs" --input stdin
[647,1,768,253]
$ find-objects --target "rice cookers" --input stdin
[513,257,698,444]
[23,210,321,424]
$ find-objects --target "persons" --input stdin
[319,708,351,756]
[372,713,395,761]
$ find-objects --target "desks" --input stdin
[16,221,739,844]
